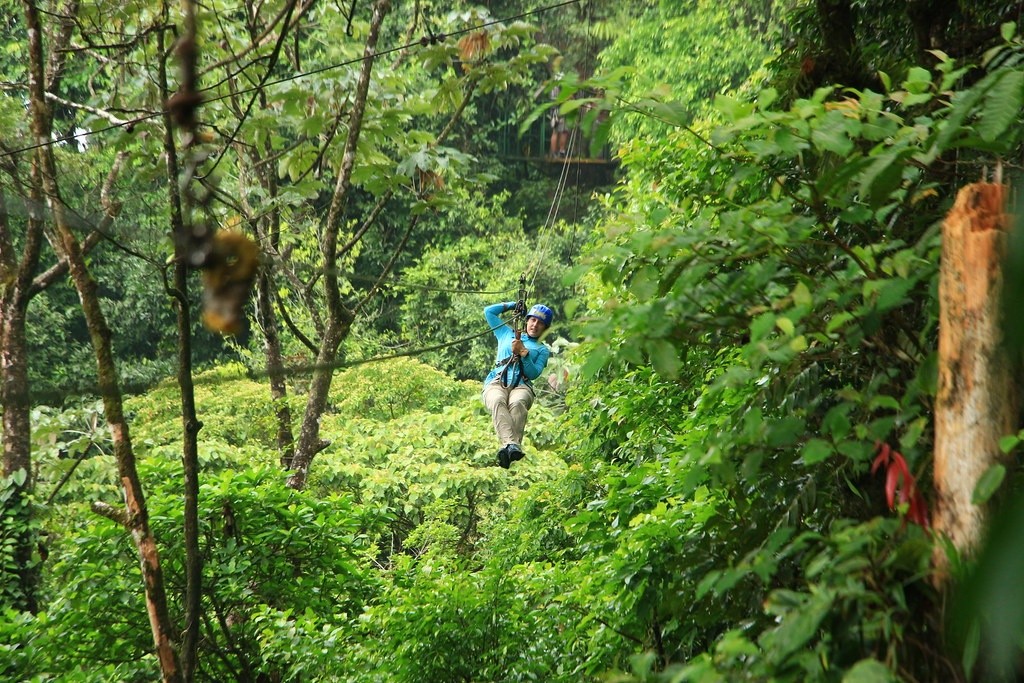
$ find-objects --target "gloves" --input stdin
[512,339,529,357]
[517,302,527,320]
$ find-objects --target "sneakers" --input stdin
[508,444,525,461]
[497,448,510,469]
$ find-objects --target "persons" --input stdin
[484,301,552,470]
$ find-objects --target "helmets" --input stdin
[526,304,553,330]
